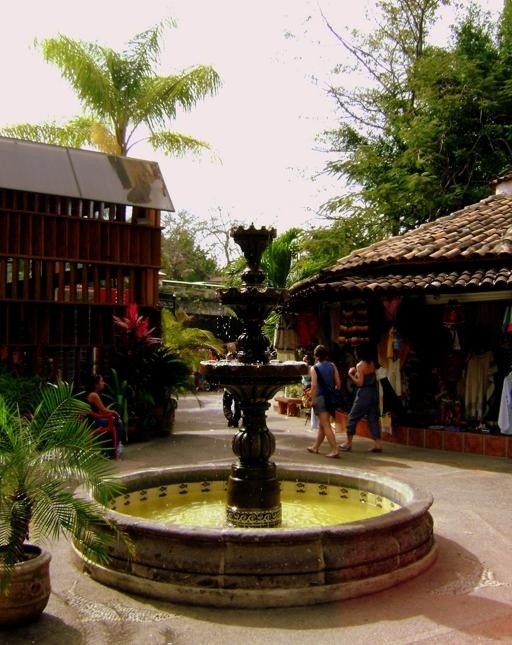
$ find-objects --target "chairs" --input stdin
[77,406,120,461]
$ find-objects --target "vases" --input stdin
[137,398,177,435]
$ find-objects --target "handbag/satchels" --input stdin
[324,389,345,409]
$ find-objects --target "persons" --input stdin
[304,344,344,459]
[222,345,245,428]
[336,344,383,454]
[298,352,317,432]
[226,342,237,363]
[78,372,126,462]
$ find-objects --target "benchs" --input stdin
[275,397,303,416]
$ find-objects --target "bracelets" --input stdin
[118,415,121,418]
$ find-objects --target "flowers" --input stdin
[111,303,202,406]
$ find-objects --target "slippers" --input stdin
[307,443,382,458]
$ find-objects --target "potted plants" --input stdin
[0,371,128,628]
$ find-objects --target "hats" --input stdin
[314,344,332,357]
